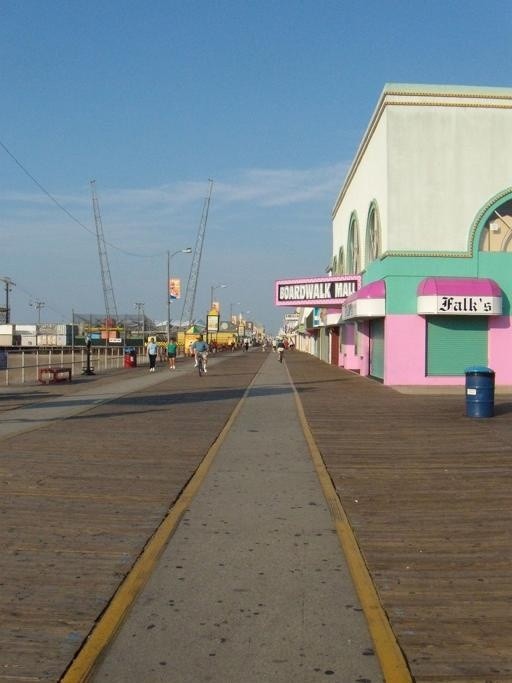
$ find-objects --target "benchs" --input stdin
[37,366,73,384]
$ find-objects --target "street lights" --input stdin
[29,300,45,323]
[133,301,147,336]
[167,248,192,345]
[210,284,257,331]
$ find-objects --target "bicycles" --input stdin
[278,347,284,363]
[192,351,208,376]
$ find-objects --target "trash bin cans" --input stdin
[124,352,136,369]
[465,367,496,420]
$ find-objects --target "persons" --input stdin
[166,338,178,370]
[210,334,295,364]
[147,337,158,372]
[190,333,210,372]
